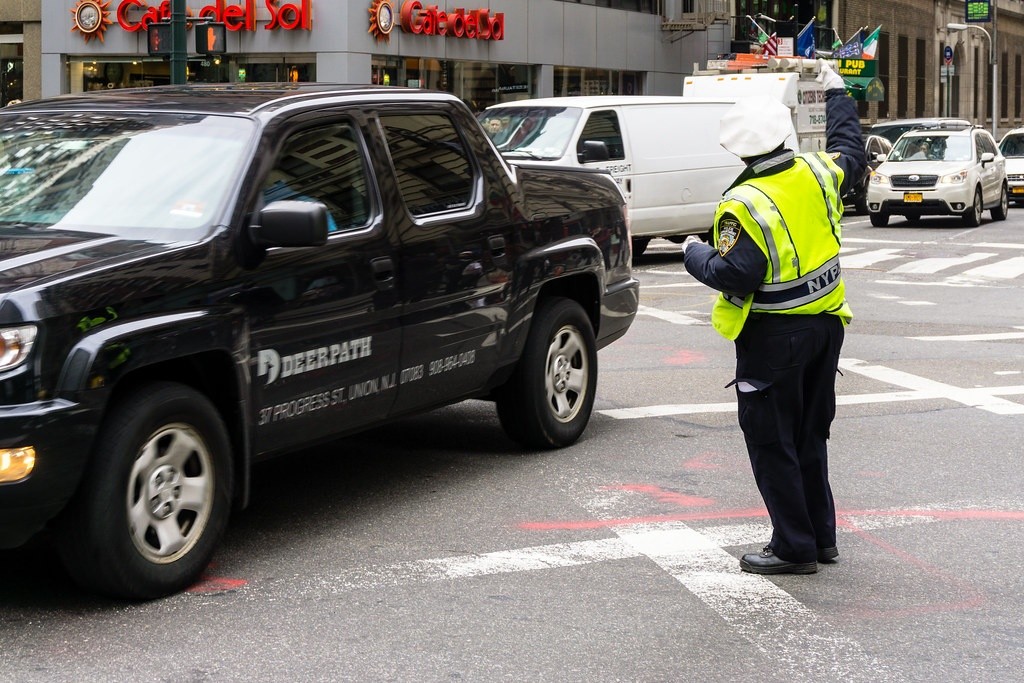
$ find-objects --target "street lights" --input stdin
[947,0,999,143]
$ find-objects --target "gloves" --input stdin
[815,58,846,92]
[680,234,709,252]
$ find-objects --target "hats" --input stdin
[719,97,796,157]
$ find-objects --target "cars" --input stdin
[0,82,640,603]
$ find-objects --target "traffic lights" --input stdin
[147,22,172,55]
[195,22,227,55]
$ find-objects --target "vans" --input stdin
[477,95,801,261]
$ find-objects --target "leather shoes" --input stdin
[815,544,839,560]
[739,543,817,575]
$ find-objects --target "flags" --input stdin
[748,27,778,58]
[796,16,817,59]
[829,24,884,59]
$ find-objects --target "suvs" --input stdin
[841,117,1024,227]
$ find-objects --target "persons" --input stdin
[680,56,856,577]
[480,115,511,141]
[915,138,936,160]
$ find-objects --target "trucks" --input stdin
[682,57,839,162]
